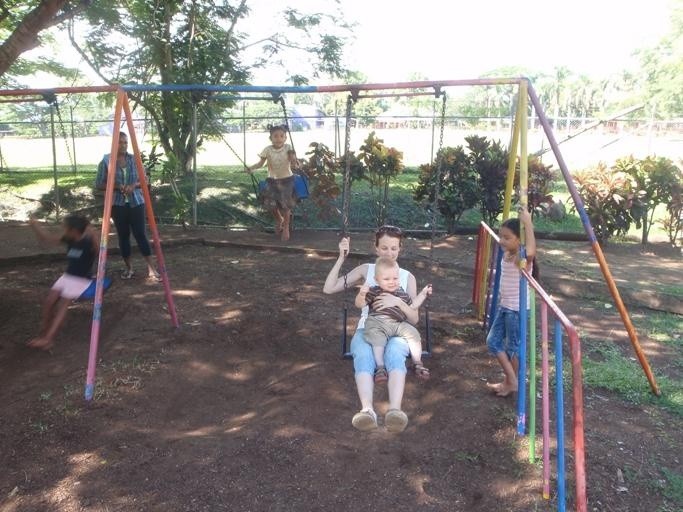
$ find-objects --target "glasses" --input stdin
[379,227,400,233]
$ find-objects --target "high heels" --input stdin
[486,381,518,398]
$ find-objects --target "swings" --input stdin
[0,94,111,298]
[192,91,308,202]
[342,90,446,360]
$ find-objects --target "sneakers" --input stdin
[384,410,408,432]
[121,270,134,280]
[143,270,162,282]
[351,409,377,431]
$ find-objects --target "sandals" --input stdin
[411,361,430,380]
[374,366,387,384]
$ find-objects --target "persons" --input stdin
[26,215,99,352]
[484,205,539,398]
[353,257,434,385]
[94,131,163,281]
[244,124,295,243]
[321,224,420,434]
